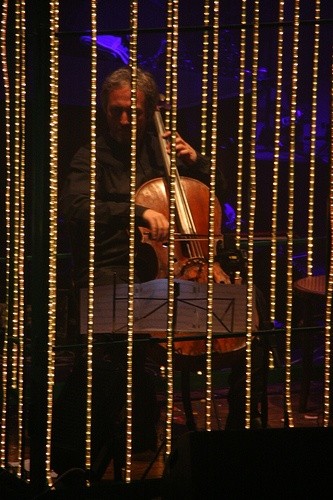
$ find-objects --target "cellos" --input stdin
[135,95,259,432]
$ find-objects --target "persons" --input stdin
[55,65,270,459]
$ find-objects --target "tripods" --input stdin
[76,276,255,480]
